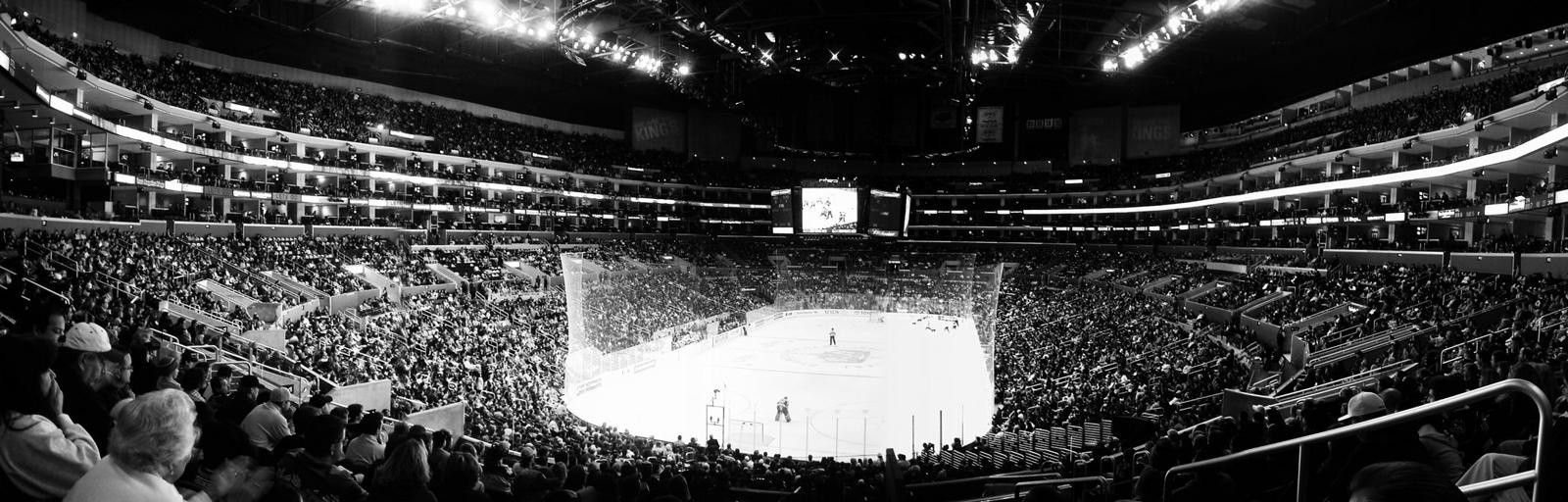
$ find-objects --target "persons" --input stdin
[0,2,1568,501]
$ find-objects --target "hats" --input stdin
[309,393,334,409]
[158,357,178,378]
[511,469,558,501]
[271,387,299,403]
[181,351,198,362]
[63,322,125,363]
[240,375,265,390]
[521,447,535,457]
